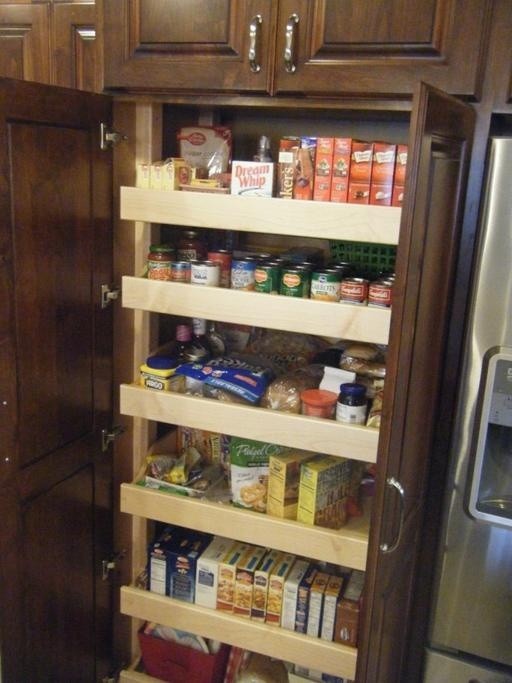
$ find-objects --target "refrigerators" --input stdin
[421,134,512,683]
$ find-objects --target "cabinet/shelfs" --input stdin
[0,0,512,683]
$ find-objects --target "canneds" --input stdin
[168,258,189,281]
[301,389,337,420]
[255,263,273,293]
[177,231,202,259]
[208,250,231,287]
[238,249,395,283]
[145,243,181,281]
[368,281,392,306]
[311,270,340,303]
[229,257,257,291]
[280,265,310,298]
[340,277,368,303]
[191,263,220,284]
[334,382,366,424]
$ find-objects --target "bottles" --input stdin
[146,231,200,284]
[299,389,337,421]
[335,382,368,425]
[173,315,208,398]
[253,135,273,161]
